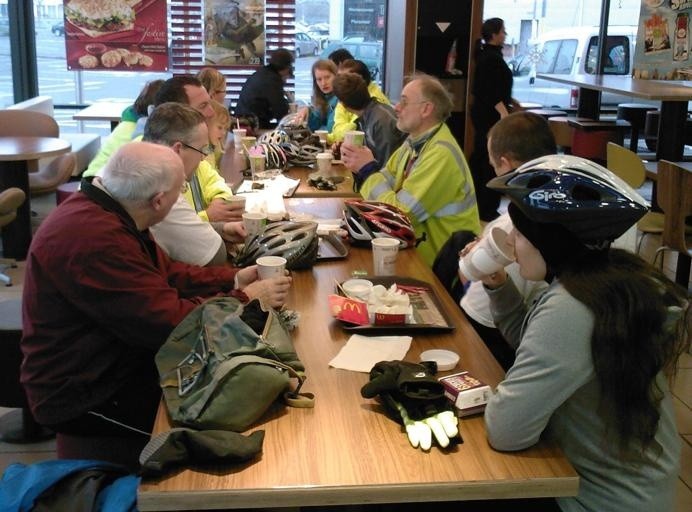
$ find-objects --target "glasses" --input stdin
[149,179,189,201]
[400,100,429,108]
[183,141,210,161]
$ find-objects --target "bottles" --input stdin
[570,88,578,108]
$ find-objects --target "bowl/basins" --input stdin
[343,280,372,295]
[421,350,460,371]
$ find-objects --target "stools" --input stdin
[511,103,692,166]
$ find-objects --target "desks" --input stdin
[234,164,360,197]
[137,198,580,512]
[73,100,135,134]
[536,75,692,162]
[0,137,72,261]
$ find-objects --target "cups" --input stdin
[370,238,401,276]
[233,130,247,151]
[459,227,517,282]
[243,137,256,156]
[250,155,265,175]
[243,213,266,235]
[315,153,333,176]
[345,130,364,148]
[315,131,328,141]
[255,257,287,281]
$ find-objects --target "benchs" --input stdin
[7,95,100,177]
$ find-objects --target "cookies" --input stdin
[78,55,98,68]
[100,50,121,68]
[118,46,153,67]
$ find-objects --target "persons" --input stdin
[457,149,692,512]
[75,64,248,269]
[233,44,408,162]
[337,69,481,288]
[469,15,521,162]
[18,138,293,461]
[453,105,559,336]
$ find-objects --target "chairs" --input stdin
[650,160,692,267]
[0,188,26,286]
[606,160,692,255]
[0,110,77,194]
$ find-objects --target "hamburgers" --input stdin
[65,1,135,38]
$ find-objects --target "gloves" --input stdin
[361,359,459,451]
[306,177,345,190]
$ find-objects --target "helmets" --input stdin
[253,112,322,169]
[235,220,318,266]
[343,198,417,249]
[487,154,652,239]
[230,113,259,135]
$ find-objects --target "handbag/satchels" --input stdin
[432,230,479,303]
[155,296,307,430]
